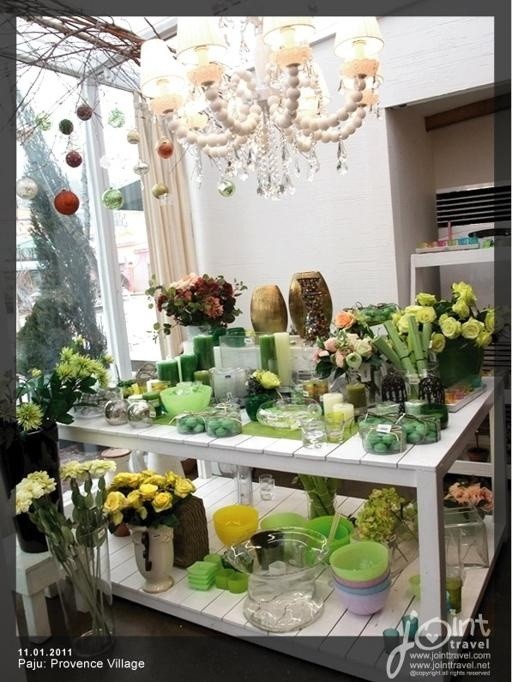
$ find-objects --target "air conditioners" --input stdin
[433,178,511,450]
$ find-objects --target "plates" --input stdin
[187,561,216,593]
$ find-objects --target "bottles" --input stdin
[235,464,253,508]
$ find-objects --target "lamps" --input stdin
[135,15,387,199]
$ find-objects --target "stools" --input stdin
[9,532,95,645]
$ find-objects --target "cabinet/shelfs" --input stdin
[56,368,511,682]
[408,244,512,484]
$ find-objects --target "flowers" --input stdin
[147,269,238,335]
[302,313,383,382]
[9,456,115,647]
[2,335,116,430]
[396,282,507,355]
[104,467,199,524]
[353,478,495,578]
[241,368,282,397]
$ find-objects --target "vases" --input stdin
[245,389,274,422]
[438,336,484,388]
[125,524,177,596]
[358,354,384,404]
[45,526,117,657]
[0,424,62,551]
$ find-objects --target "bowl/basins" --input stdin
[256,398,323,433]
[330,542,391,617]
[216,505,353,565]
[407,575,421,598]
[159,385,213,414]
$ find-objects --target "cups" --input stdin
[209,365,246,408]
[259,475,275,501]
[322,392,354,425]
[203,553,247,596]
[150,380,170,392]
[346,382,368,415]
[301,417,324,448]
[326,412,345,442]
[446,567,463,615]
